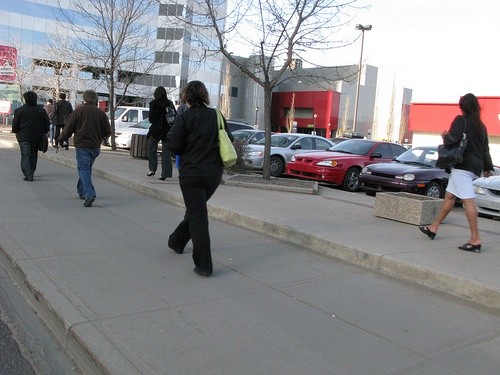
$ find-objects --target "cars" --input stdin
[342,131,364,139]
[463,175,500,219]
[242,133,336,177]
[330,138,349,145]
[285,139,409,192]
[231,129,274,145]
[358,146,500,198]
[109,118,152,150]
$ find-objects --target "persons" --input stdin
[56,90,111,207]
[43,99,54,146]
[177,92,190,118]
[146,86,178,181]
[418,93,494,253]
[12,91,51,181]
[166,81,234,277]
[49,93,73,150]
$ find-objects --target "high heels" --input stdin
[147,172,155,176]
[419,226,437,239]
[159,176,168,180]
[458,241,481,253]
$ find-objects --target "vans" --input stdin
[104,106,150,146]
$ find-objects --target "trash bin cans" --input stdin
[130,134,147,158]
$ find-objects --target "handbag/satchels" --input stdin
[435,114,468,169]
[215,107,237,168]
[38,133,48,153]
[165,106,177,124]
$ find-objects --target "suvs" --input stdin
[157,119,254,160]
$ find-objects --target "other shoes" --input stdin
[24,175,33,181]
[65,147,69,150]
[53,145,59,148]
[168,239,183,254]
[79,194,85,199]
[84,196,95,207]
[194,266,213,276]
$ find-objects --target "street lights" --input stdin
[353,23,372,131]
